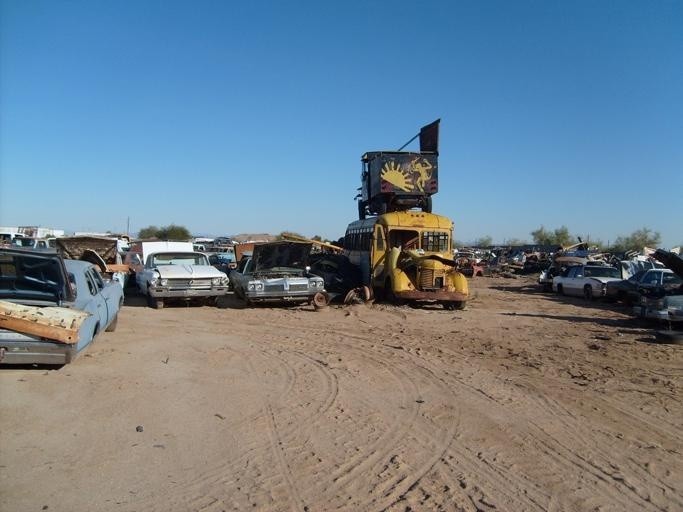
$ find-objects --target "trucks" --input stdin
[354,116,441,220]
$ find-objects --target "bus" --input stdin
[342,208,470,311]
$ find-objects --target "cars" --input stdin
[135,240,229,308]
[1,245,127,367]
[551,251,683,324]
[229,239,328,307]
